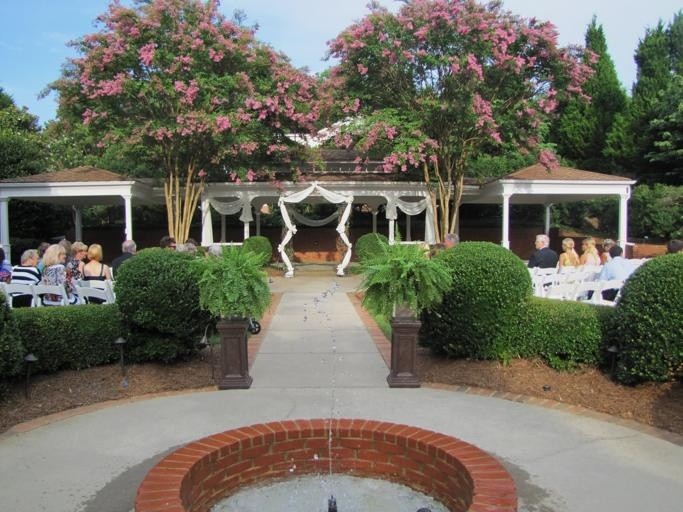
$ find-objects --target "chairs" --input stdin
[0,279,116,308]
[527,255,648,308]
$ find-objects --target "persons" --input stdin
[0,235,223,308]
[444,232,459,248]
[524,233,559,289]
[599,238,615,265]
[577,236,602,283]
[588,245,633,301]
[557,237,580,288]
[420,241,432,260]
[431,243,445,253]
[668,239,683,254]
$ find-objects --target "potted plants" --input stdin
[189,238,270,388]
[353,233,453,388]
